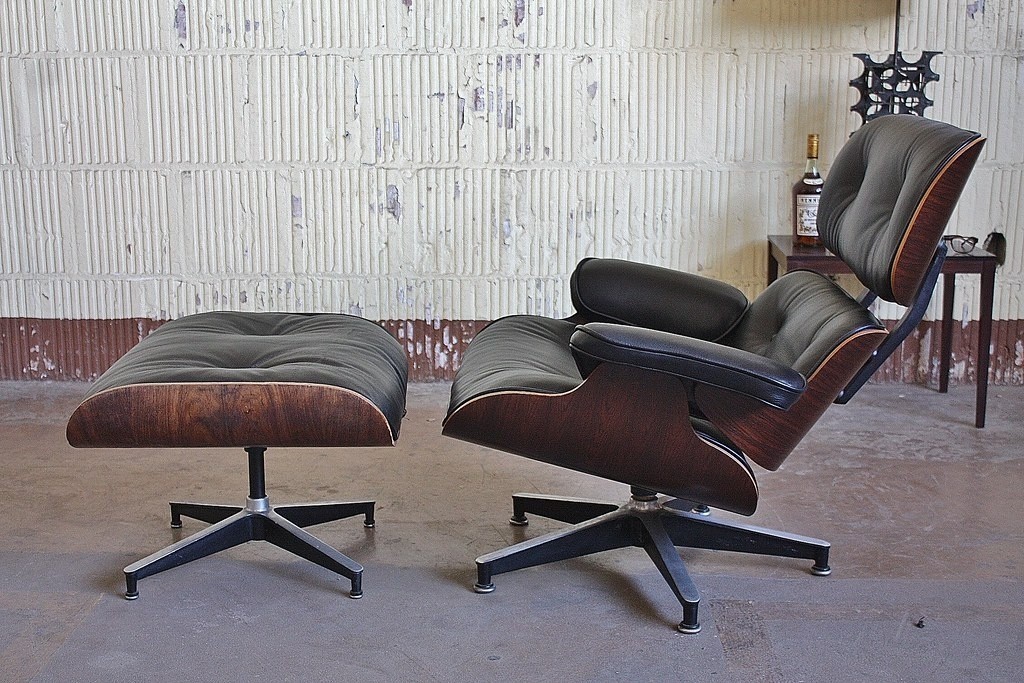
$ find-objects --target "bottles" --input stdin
[791,134,825,249]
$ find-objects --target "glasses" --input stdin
[942,235,978,254]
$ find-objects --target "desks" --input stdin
[767,233,998,429]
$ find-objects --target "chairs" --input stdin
[445,114,987,636]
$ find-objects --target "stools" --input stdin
[64,309,410,603]
